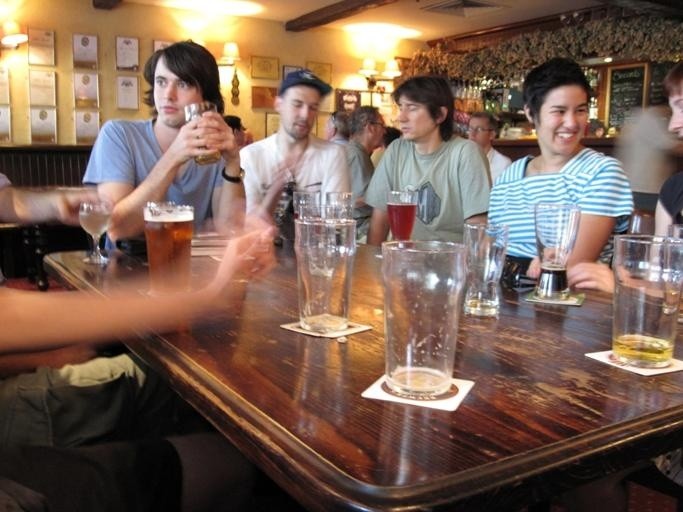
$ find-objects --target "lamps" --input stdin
[2,20,28,46]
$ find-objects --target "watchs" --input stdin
[221,166,246,183]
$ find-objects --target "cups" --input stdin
[293,217,357,334]
[609,232,683,370]
[142,200,195,297]
[378,240,469,400]
[531,201,582,302]
[182,100,224,166]
[325,192,356,222]
[291,190,325,234]
[384,189,420,241]
[461,222,509,319]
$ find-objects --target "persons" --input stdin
[0,227,278,511]
[488,57,633,274]
[223,114,245,150]
[612,93,683,216]
[0,171,113,225]
[370,124,400,172]
[239,70,352,223]
[344,106,387,228]
[362,76,491,248]
[326,110,350,149]
[565,60,683,294]
[466,111,512,190]
[83,41,248,243]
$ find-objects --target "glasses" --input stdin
[467,125,494,134]
[369,118,385,128]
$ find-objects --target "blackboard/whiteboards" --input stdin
[606,62,649,134]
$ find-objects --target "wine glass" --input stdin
[78,188,115,266]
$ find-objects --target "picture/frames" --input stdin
[73,73,99,110]
[72,33,98,69]
[116,76,139,111]
[0,106,11,145]
[74,111,100,145]
[29,70,56,107]
[305,62,332,86]
[265,112,280,138]
[28,28,55,67]
[153,40,173,53]
[30,107,58,144]
[250,56,280,79]
[251,86,279,111]
[115,35,139,71]
[0,67,10,105]
[282,65,302,80]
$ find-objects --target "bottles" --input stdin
[452,79,485,114]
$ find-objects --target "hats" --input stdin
[278,71,331,98]
